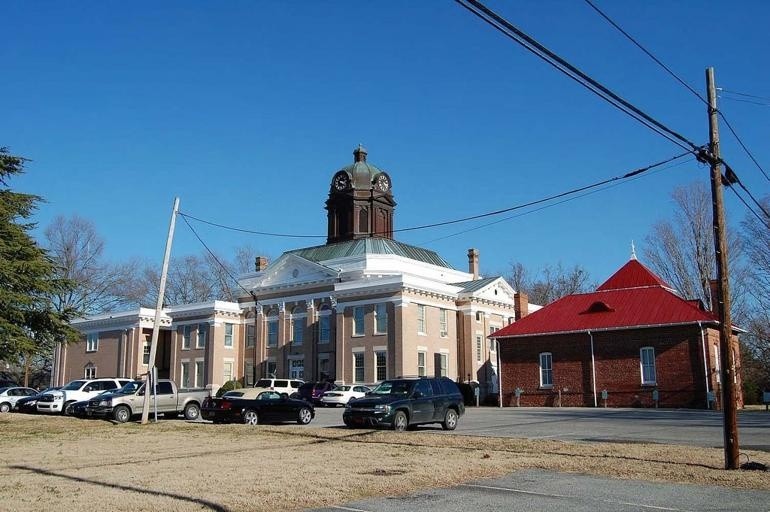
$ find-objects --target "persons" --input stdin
[708,388,716,409]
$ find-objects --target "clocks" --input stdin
[373,171,392,193]
[331,170,352,192]
